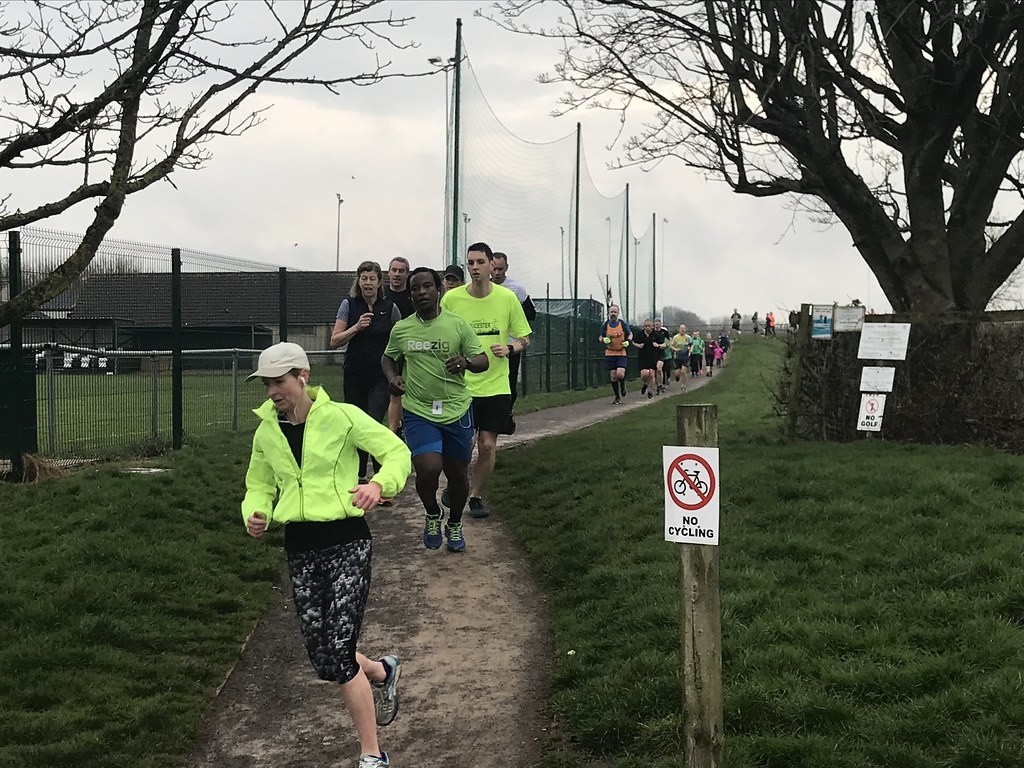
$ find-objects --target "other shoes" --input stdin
[656,388,660,395]
[359,476,368,484]
[676,376,679,382]
[611,398,621,406]
[667,378,670,385]
[378,495,394,506]
[706,372,710,377]
[621,385,626,397]
[640,385,648,394]
[647,390,653,399]
[662,387,666,392]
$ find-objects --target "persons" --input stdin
[731,308,742,331]
[869,308,876,315]
[752,312,759,333]
[330,243,537,552]
[789,310,801,330]
[241,342,412,768]
[764,312,776,337]
[598,305,731,405]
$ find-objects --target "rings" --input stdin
[457,365,460,368]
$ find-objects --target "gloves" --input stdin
[621,341,629,347]
[603,337,611,344]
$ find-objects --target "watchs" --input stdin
[465,356,472,366]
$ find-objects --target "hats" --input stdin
[244,342,310,382]
[443,265,464,281]
[661,327,668,331]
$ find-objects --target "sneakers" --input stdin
[441,488,451,508]
[370,654,402,726]
[444,518,466,552]
[424,504,444,549]
[358,751,390,768]
[468,495,489,518]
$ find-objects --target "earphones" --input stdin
[438,291,441,299]
[300,377,306,386]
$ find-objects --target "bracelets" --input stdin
[507,344,515,353]
